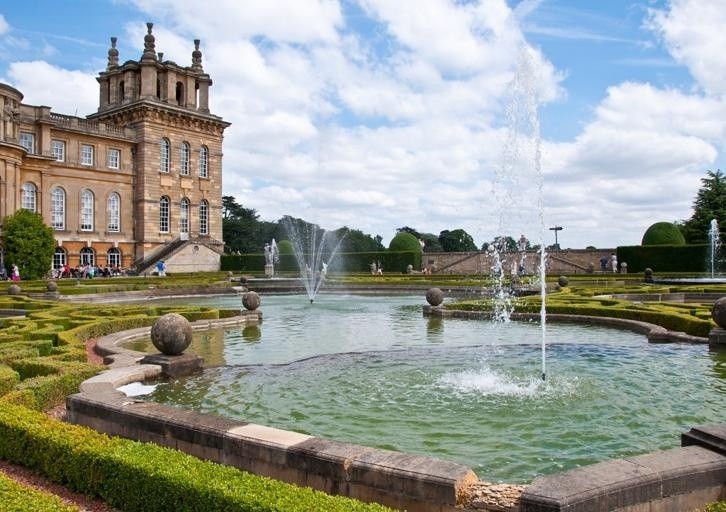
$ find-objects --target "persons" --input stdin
[264,242,272,264]
[10,263,21,280]
[610,252,619,273]
[161,260,167,277]
[600,255,608,271]
[422,265,433,274]
[377,260,384,275]
[305,259,313,274]
[0,263,7,280]
[157,259,163,277]
[371,260,376,275]
[320,259,328,275]
[58,263,128,281]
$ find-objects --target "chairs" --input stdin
[56,266,125,278]
